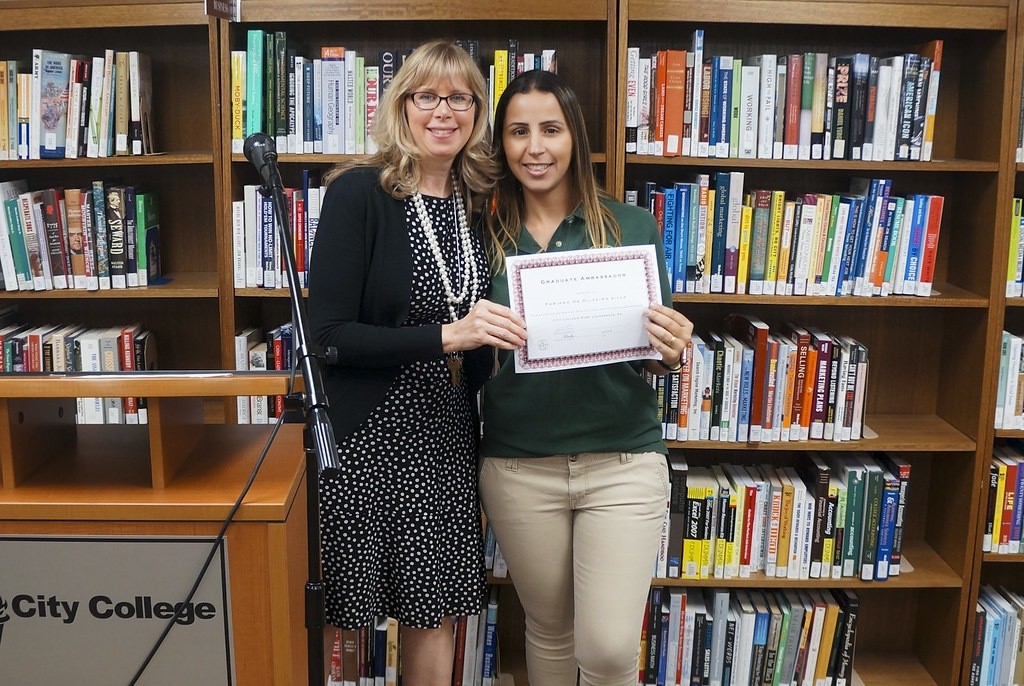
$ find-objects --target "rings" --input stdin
[668,336,676,347]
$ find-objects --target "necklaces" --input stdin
[404,163,477,322]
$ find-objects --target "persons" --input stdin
[477,69,694,686]
[302,41,530,685]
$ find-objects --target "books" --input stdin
[0,30,1023,686]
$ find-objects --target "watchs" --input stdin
[657,347,687,371]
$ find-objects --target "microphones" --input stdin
[243,133,285,198]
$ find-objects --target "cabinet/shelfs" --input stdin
[0,0,1024,686]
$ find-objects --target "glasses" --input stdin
[408,92,475,111]
